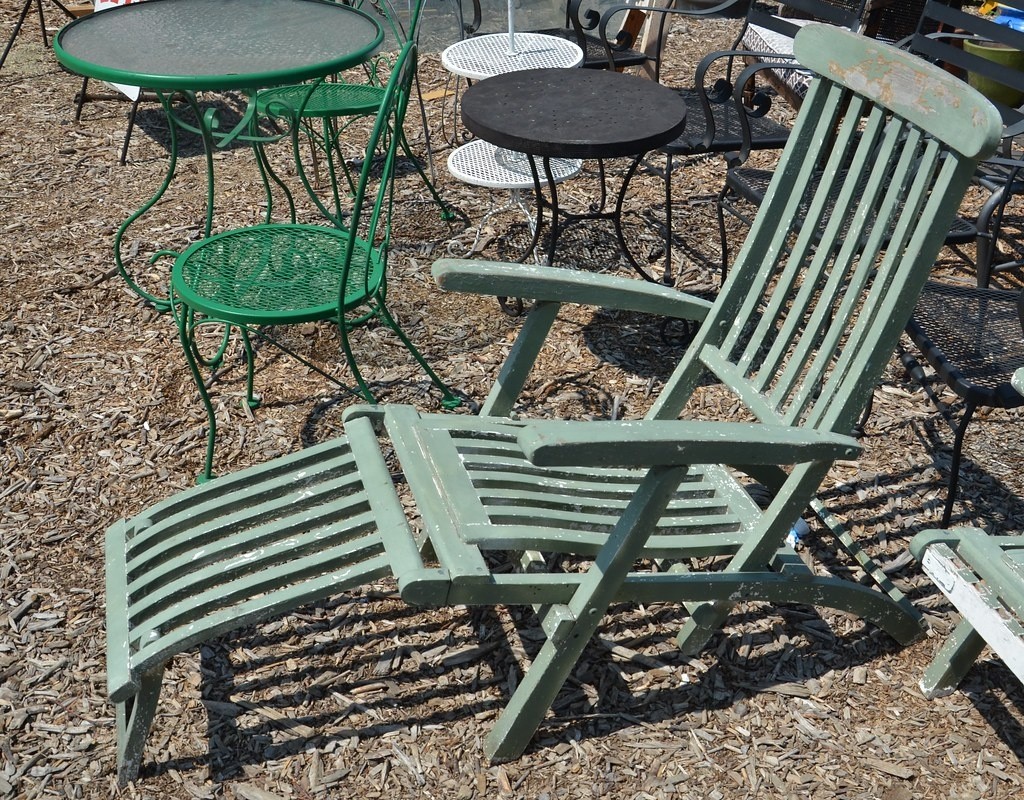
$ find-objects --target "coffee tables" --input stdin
[53,0,388,332]
[461,68,688,317]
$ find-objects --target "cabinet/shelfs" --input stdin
[442,0,582,266]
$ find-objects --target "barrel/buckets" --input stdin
[963,33,1024,110]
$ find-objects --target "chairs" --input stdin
[850,152,1024,531]
[170,40,461,485]
[256,0,455,231]
[909,365,1024,700]
[590,0,873,288]
[716,0,1024,403]
[474,0,676,86]
[105,22,1004,785]
